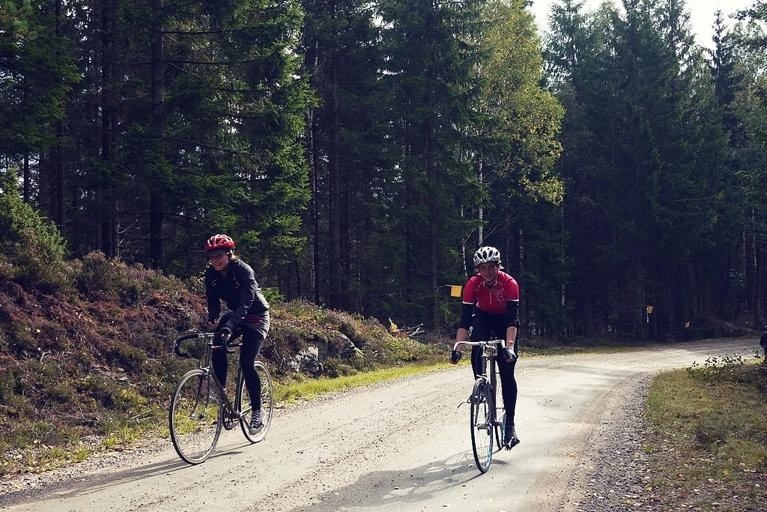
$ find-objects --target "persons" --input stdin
[202,233,271,436]
[449,246,520,447]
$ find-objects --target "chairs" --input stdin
[505,425,516,449]
[249,407,265,435]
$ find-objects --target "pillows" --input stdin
[473,246,500,267]
[206,233,235,250]
